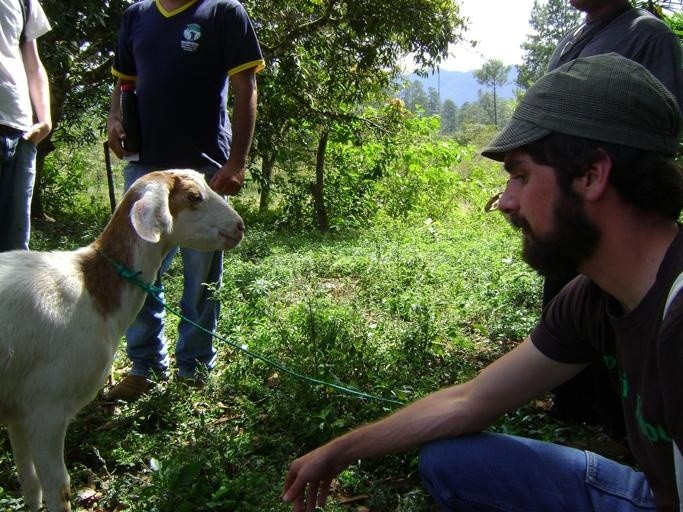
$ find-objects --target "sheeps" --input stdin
[0,168,246,512]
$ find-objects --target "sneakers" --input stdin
[98,375,158,406]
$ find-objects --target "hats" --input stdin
[481,51,683,163]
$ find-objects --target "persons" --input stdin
[546,2,683,438]
[0,0,56,253]
[280,51,682,511]
[107,0,267,405]
